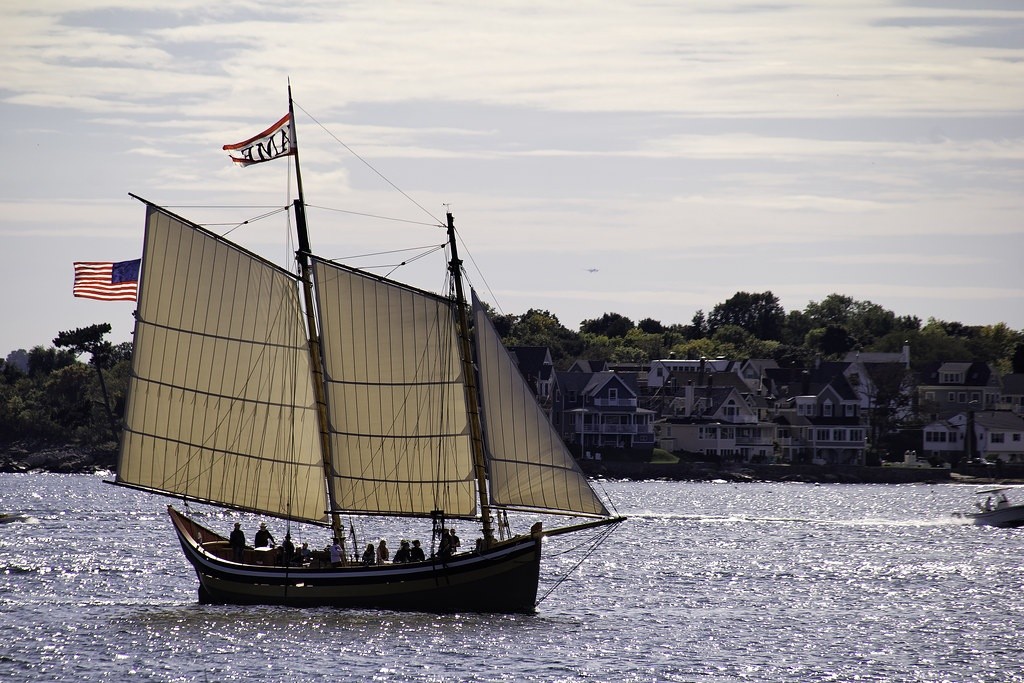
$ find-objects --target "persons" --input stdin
[362,544,375,565]
[275,534,311,567]
[376,540,389,563]
[449,529,460,554]
[393,540,425,562]
[230,523,245,558]
[255,523,277,548]
[329,537,343,567]
[994,494,1009,510]
[985,497,991,512]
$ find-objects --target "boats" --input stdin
[950,486,1024,529]
[949,457,1024,484]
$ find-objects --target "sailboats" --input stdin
[102,77,628,617]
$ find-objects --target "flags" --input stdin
[73,258,141,302]
[223,113,295,168]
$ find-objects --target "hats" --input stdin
[260,523,267,527]
[302,542,308,546]
[234,523,241,527]
